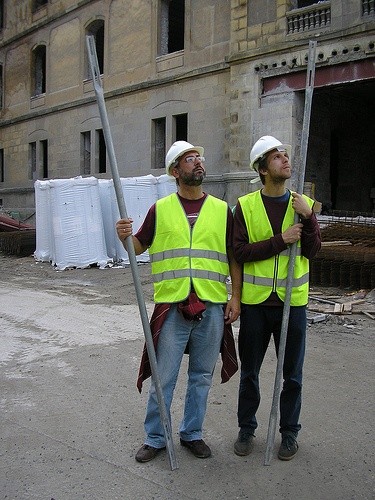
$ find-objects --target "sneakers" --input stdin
[278,431,299,461]
[135,442,166,463]
[180,437,211,458]
[234,432,257,457]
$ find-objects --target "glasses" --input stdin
[185,156,205,163]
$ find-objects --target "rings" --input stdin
[123,228,126,233]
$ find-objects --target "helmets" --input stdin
[165,141,204,175]
[250,135,292,185]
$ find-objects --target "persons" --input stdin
[115,141,243,462]
[231,135,322,460]
